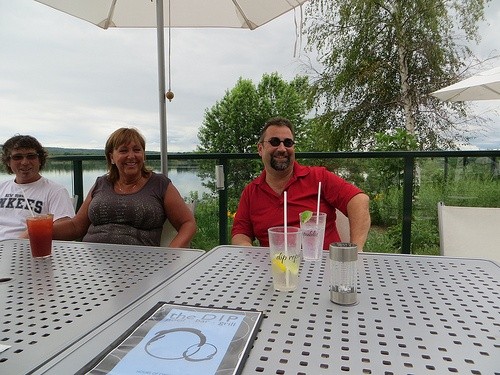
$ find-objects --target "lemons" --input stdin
[271,254,287,272]
[302,210,313,223]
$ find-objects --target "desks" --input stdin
[0,238,206,375]
[29,245,500,375]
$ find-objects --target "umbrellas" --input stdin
[35,0,307,177]
[428,67,500,102]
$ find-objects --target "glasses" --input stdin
[259,137,295,147]
[10,154,39,161]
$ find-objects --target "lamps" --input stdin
[215,165,225,190]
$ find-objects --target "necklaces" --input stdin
[117,181,136,194]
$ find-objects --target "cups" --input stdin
[26,214,54,259]
[267,226,302,292]
[299,211,327,262]
[328,242,358,306]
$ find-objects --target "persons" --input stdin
[20,128,197,248]
[232,117,371,251]
[0,133,75,241]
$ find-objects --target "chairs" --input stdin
[159,204,194,249]
[335,208,350,244]
[438,201,500,263]
